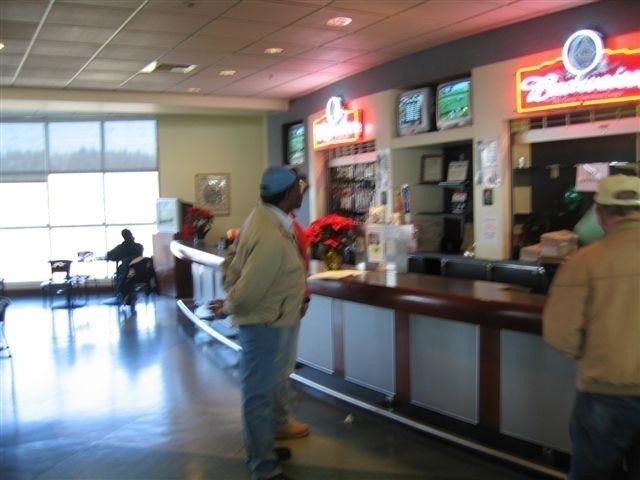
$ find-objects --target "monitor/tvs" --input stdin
[435,76,470,130]
[395,87,433,136]
[285,122,305,165]
[153,197,193,235]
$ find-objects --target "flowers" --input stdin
[181,206,215,240]
[303,212,363,259]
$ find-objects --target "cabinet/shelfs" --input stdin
[417,140,473,252]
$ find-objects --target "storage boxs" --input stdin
[364,222,415,272]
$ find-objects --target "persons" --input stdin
[273,164,310,440]
[108,229,143,303]
[209,167,308,480]
[542,175,640,479]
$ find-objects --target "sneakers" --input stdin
[272,446,291,460]
[273,421,311,440]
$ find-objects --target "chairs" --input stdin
[40,254,155,311]
[0,295,12,359]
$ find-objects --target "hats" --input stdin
[259,163,297,197]
[593,173,640,206]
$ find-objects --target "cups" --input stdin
[386,264,397,287]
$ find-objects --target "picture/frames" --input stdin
[194,173,230,216]
[421,155,446,184]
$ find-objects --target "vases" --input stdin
[194,236,201,244]
[326,251,343,270]
